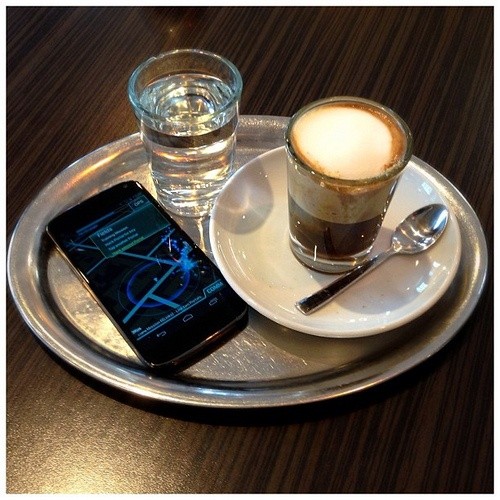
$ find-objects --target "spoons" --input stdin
[294,203,450,315]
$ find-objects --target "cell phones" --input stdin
[42,180,250,377]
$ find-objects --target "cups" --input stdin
[127,49,243,217]
[286,95,413,274]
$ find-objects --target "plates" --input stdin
[209,144,463,340]
